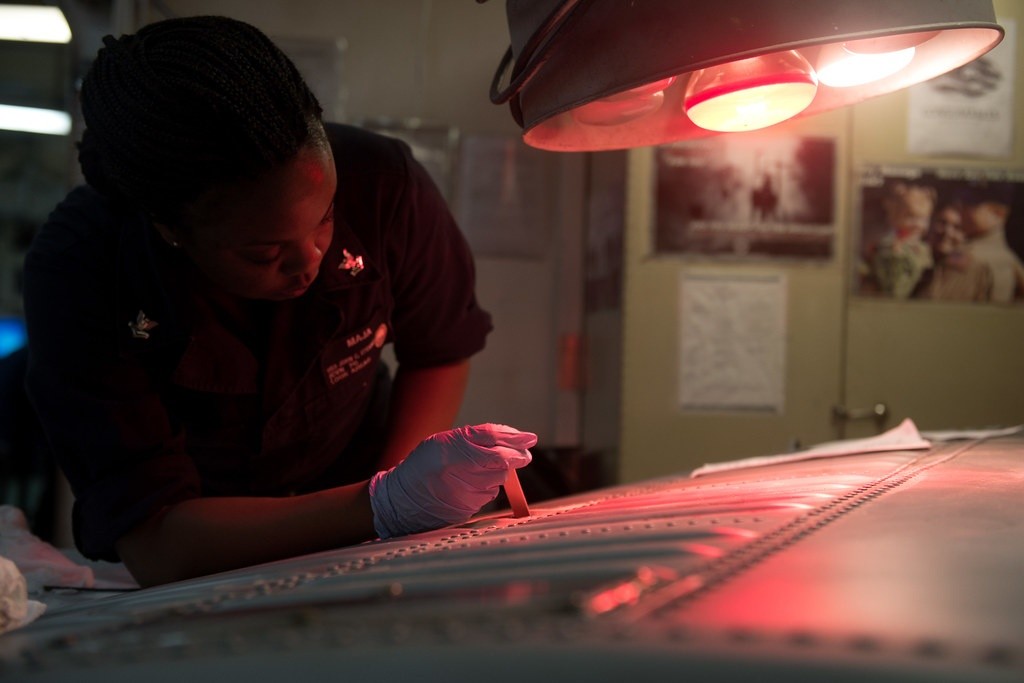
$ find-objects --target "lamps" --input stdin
[492,0,1007,154]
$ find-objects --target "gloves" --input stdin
[369,424,538,539]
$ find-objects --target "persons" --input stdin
[15,14,539,574]
[858,178,1024,305]
